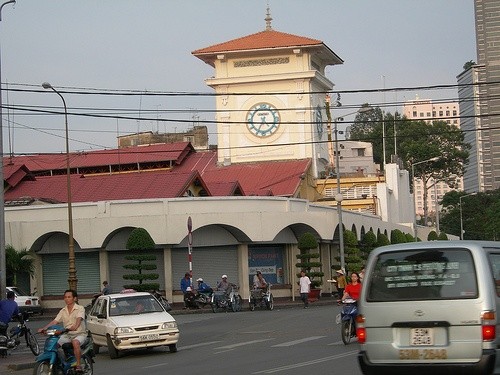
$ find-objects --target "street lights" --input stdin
[412,156,441,242]
[42,82,78,305]
[460,193,477,240]
[334,107,373,278]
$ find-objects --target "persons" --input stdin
[253,271,270,291]
[0,291,21,338]
[134,300,148,313]
[332,270,347,306]
[196,278,210,293]
[299,271,311,309]
[184,287,198,310]
[92,281,112,298]
[38,289,88,373]
[218,274,232,294]
[181,273,194,308]
[341,272,362,300]
[359,267,366,283]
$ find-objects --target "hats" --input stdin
[336,270,343,274]
[186,287,192,291]
[196,278,203,282]
[221,275,228,279]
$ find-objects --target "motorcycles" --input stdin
[336,297,359,345]
[121,289,172,312]
[0,311,40,356]
[32,325,96,375]
[184,285,242,313]
[249,282,274,312]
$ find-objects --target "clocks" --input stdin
[241,92,287,143]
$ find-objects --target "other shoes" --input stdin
[304,305,308,308]
[350,332,355,337]
[76,366,82,371]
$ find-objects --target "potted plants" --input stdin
[296,232,321,303]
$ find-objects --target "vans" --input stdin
[355,240,500,375]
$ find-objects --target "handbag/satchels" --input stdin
[335,314,342,325]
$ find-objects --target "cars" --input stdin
[86,292,179,359]
[6,287,41,322]
